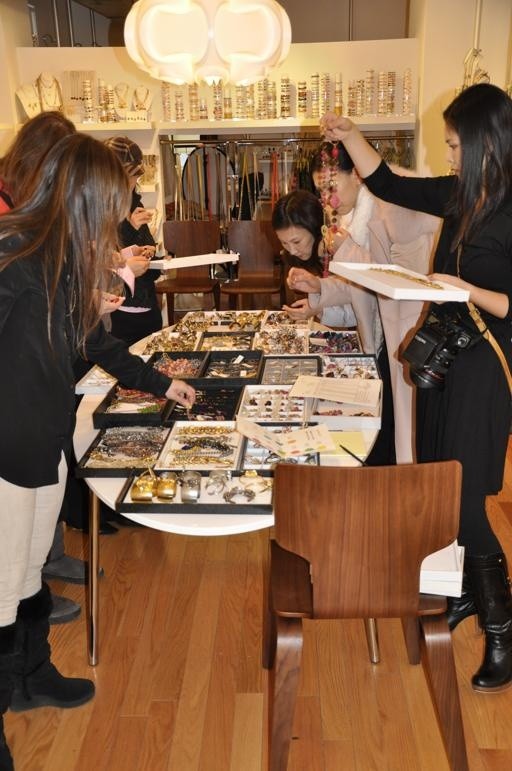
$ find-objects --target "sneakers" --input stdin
[65,512,142,534]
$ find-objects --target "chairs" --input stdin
[151,220,288,326]
[258,462,471,771]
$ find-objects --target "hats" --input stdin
[104,135,145,177]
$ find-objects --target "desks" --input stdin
[72,309,386,668]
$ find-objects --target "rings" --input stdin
[321,363,374,379]
[249,390,303,418]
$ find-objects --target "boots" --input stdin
[41,523,103,584]
[1,583,94,771]
[448,545,511,695]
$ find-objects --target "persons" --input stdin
[320,84,511,692]
[288,140,445,465]
[1,134,195,771]
[272,190,357,327]
[1,113,176,621]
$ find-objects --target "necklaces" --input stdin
[114,85,149,111]
[89,353,255,468]
[320,133,338,278]
[375,268,443,291]
[21,79,63,111]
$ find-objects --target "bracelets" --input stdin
[206,470,269,504]
[83,82,116,121]
[162,71,411,122]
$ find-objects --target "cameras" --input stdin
[403,302,481,390]
